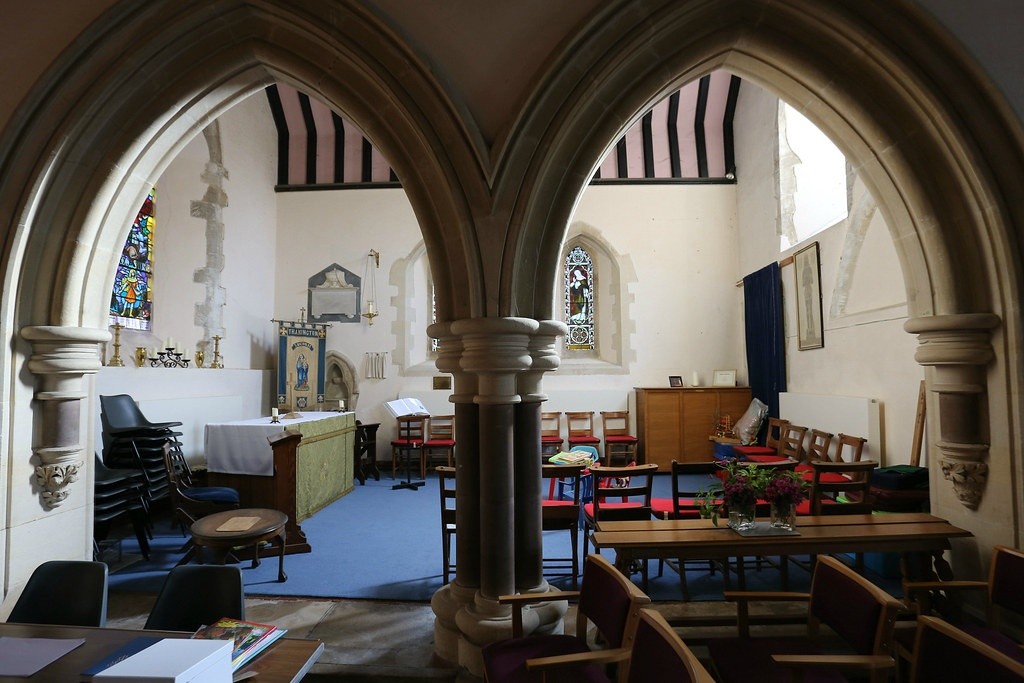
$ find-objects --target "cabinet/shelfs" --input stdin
[633,387,753,473]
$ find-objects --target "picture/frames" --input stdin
[792,241,823,351]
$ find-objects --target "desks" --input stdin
[190,509,288,582]
[204,410,357,525]
[0,623,324,683]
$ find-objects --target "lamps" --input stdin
[360,249,380,326]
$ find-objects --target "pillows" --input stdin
[733,397,769,446]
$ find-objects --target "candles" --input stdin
[339,400,344,407]
[272,408,278,416]
[151,337,189,359]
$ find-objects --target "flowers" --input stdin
[696,456,817,527]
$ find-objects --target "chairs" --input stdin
[144,565,246,632]
[92,394,240,571]
[734,417,879,591]
[541,409,658,592]
[650,460,732,604]
[6,561,109,627]
[482,550,1023,683]
[391,415,456,586]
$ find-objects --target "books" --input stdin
[548,449,595,466]
[194,615,289,674]
[383,398,430,418]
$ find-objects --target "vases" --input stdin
[727,504,756,529]
[770,503,795,529]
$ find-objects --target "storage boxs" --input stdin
[92,638,234,683]
